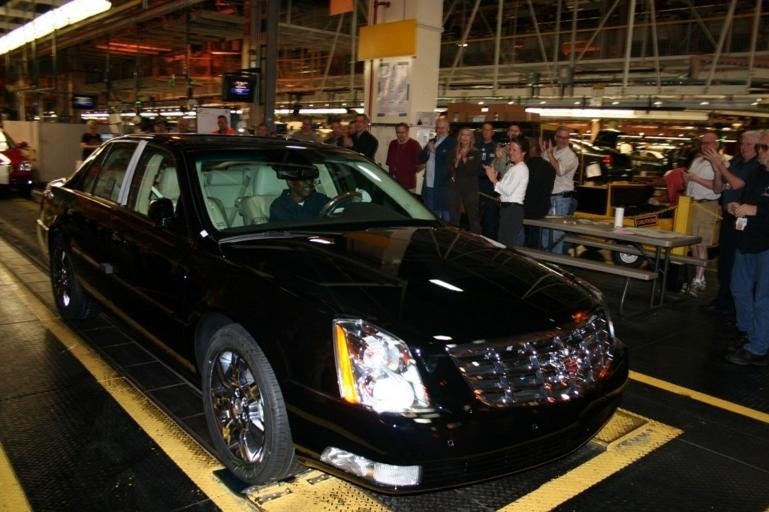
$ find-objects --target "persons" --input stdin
[80,121,102,162]
[270,163,374,231]
[383,112,580,273]
[123,114,271,135]
[677,126,769,370]
[294,112,379,162]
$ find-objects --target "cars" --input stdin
[0,121,38,198]
[37,135,631,497]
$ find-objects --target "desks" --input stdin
[520,214,702,322]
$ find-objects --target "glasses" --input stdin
[556,134,572,139]
[754,144,768,152]
[699,140,717,145]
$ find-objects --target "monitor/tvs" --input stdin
[221,75,257,104]
[71,93,99,109]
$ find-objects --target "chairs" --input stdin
[240,166,289,226]
[206,168,249,229]
[154,165,228,234]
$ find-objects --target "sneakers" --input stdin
[690,279,701,296]
[681,280,705,292]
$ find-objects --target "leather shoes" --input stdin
[730,349,767,366]
[728,343,749,358]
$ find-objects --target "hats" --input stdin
[154,115,168,127]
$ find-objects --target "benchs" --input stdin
[563,235,707,308]
[513,243,657,322]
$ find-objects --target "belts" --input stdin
[692,199,711,203]
[551,192,565,196]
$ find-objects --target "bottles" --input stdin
[552,199,556,216]
[614,207,625,229]
